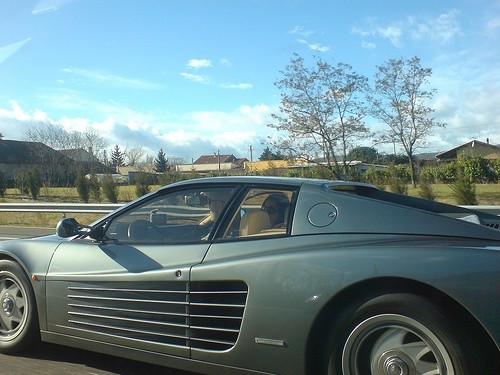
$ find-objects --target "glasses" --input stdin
[261,205,277,214]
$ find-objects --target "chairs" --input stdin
[238,210,271,237]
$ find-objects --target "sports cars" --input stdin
[0,175,500,375]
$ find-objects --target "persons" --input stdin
[261,194,290,229]
[201,191,233,241]
